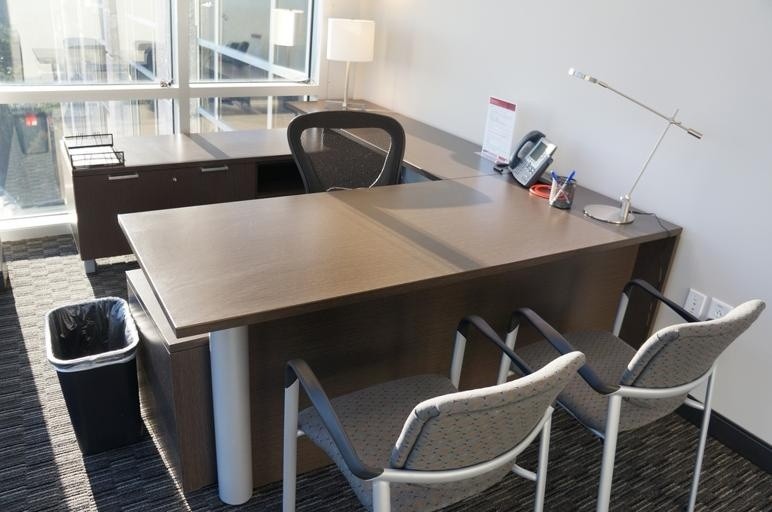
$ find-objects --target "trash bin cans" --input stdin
[45,296,143,454]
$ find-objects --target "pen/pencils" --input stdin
[549,170,575,206]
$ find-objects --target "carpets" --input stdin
[2,117,64,209]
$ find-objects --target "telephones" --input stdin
[508,130,558,187]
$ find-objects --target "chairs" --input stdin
[282,311,587,511]
[203,42,251,108]
[494,277,765,512]
[286,110,404,192]
[129,46,156,111]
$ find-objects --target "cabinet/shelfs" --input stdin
[125,244,641,494]
[55,140,256,275]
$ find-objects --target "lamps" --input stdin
[323,18,374,112]
[270,9,295,129]
[567,66,704,224]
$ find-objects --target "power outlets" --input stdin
[706,297,733,322]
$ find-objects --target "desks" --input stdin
[116,174,683,506]
[285,98,519,181]
